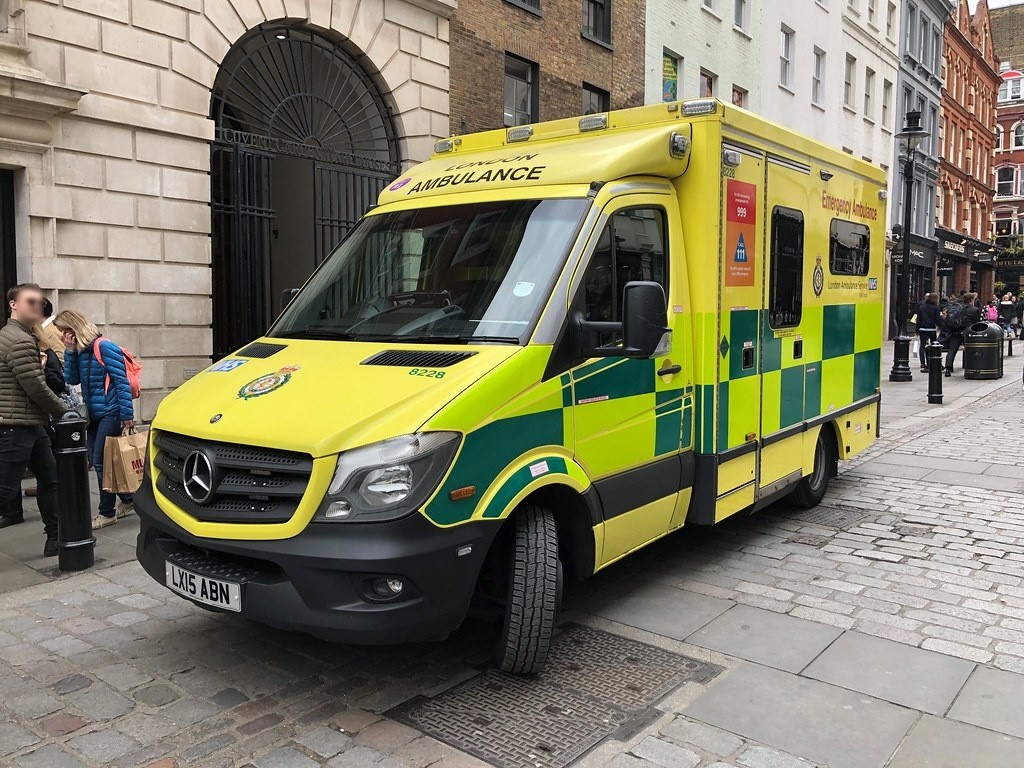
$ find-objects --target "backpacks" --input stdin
[93,337,142,399]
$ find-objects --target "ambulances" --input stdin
[132,96,889,682]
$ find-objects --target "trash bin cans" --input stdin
[964,322,1004,378]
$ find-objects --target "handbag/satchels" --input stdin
[102,426,149,493]
[49,367,85,421]
[913,333,918,358]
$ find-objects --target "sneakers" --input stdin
[115,502,134,518]
[92,513,118,531]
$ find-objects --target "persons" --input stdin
[55,310,137,529]
[915,293,946,373]
[949,294,958,301]
[945,293,979,377]
[32,296,67,369]
[956,289,966,304]
[0,283,97,555]
[916,292,930,314]
[25,322,66,496]
[973,291,981,320]
[937,296,955,352]
[980,291,1024,340]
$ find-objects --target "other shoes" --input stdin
[44,538,59,557]
[0,516,25,530]
[945,370,951,377]
[921,364,926,368]
[24,486,37,496]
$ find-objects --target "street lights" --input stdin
[888,108,932,382]
